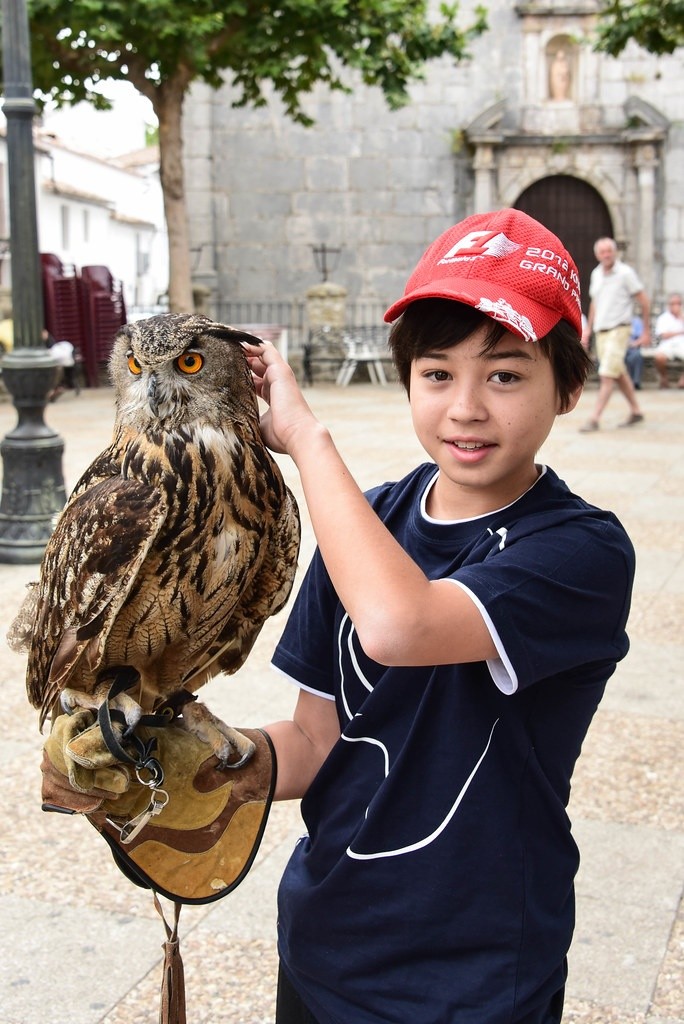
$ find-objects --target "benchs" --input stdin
[588,352,684,366]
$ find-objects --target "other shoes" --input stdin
[620,414,644,427]
[579,421,599,433]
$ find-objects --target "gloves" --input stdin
[41,709,278,1024]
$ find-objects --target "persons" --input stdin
[40,209,636,1024]
[41,329,84,398]
[625,315,644,389]
[580,238,653,433]
[654,293,684,388]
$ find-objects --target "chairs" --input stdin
[41,253,126,389]
[335,327,387,387]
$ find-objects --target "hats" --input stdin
[384,206,582,344]
